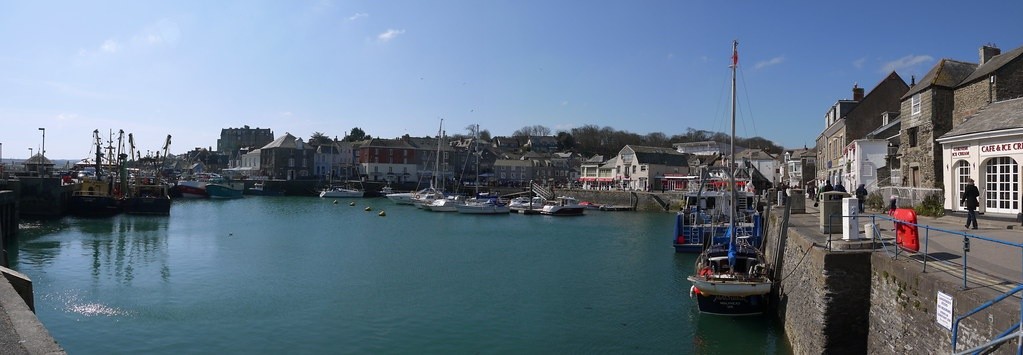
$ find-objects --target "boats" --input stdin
[380,123,586,216]
[18,128,175,219]
[177,180,244,198]
[673,166,762,252]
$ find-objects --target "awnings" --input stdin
[598,177,614,182]
[579,177,585,182]
[585,177,596,182]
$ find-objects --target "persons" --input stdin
[815,179,834,199]
[888,196,896,215]
[786,181,801,189]
[834,181,847,200]
[962,178,981,231]
[855,183,868,213]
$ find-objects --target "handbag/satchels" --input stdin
[976,202,979,207]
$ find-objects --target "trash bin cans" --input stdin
[767,188,776,201]
[787,189,806,214]
[819,191,850,235]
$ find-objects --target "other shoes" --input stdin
[972,227,978,229]
[965,225,969,228]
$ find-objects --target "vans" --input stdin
[192,169,249,179]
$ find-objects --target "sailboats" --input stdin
[319,138,367,198]
[687,38,776,317]
